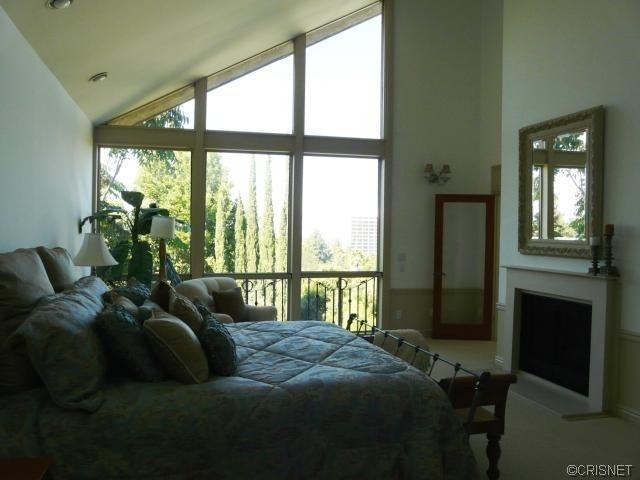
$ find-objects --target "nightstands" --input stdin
[0,455,56,480]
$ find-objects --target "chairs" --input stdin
[174,276,277,320]
[441,375,517,480]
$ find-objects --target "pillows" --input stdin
[0,247,237,413]
[212,288,246,320]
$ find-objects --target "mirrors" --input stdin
[519,103,605,259]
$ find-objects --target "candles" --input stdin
[590,237,601,246]
[605,223,614,234]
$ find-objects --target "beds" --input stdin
[0,314,489,480]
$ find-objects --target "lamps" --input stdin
[89,71,107,82]
[73,234,119,277]
[151,216,175,287]
[425,164,451,184]
[46,0,73,10]
